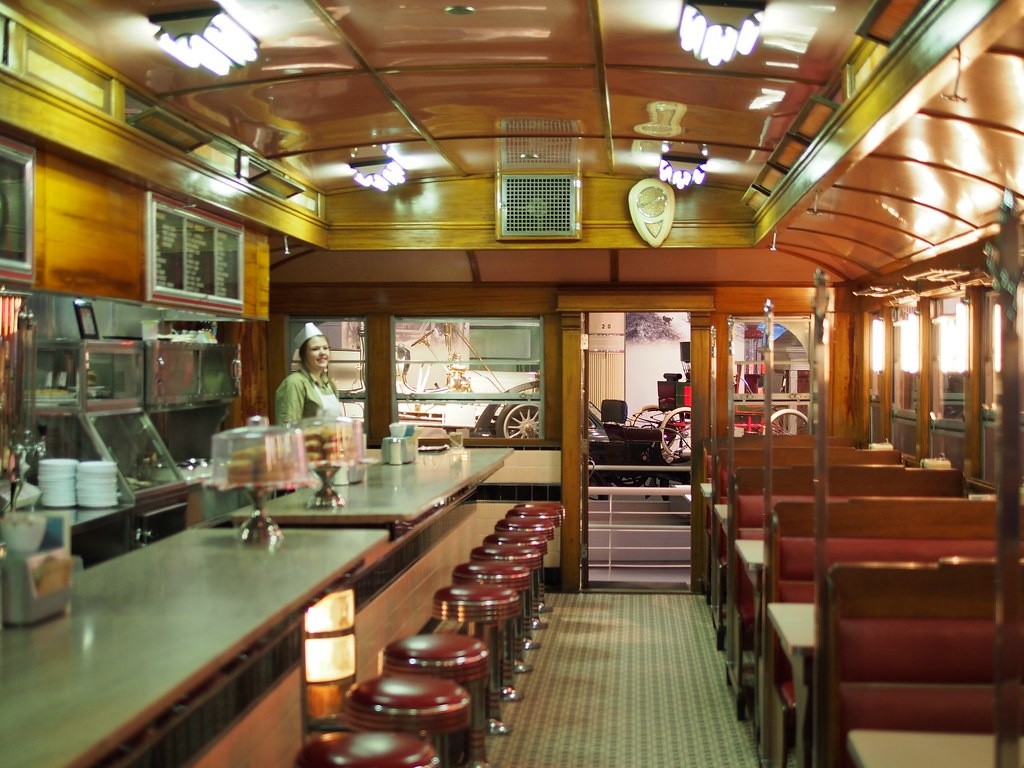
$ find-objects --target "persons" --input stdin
[273,323,342,426]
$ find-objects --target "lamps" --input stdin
[349,157,407,192]
[660,151,707,190]
[679,0,765,66]
[148,7,260,77]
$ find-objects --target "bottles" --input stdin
[389,439,402,466]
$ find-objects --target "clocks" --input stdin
[628,178,676,247]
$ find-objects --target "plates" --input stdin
[34,455,120,509]
[24,393,77,404]
[68,385,104,391]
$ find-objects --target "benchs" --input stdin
[700,441,1024,768]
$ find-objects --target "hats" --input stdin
[294,322,323,347]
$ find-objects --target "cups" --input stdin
[450,432,464,451]
[140,319,159,340]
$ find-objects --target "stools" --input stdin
[294,500,565,768]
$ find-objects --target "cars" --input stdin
[286,328,817,489]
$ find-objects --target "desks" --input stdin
[700,483,712,498]
[767,600,816,768]
[733,538,764,741]
[713,504,727,526]
[846,728,1024,768]
